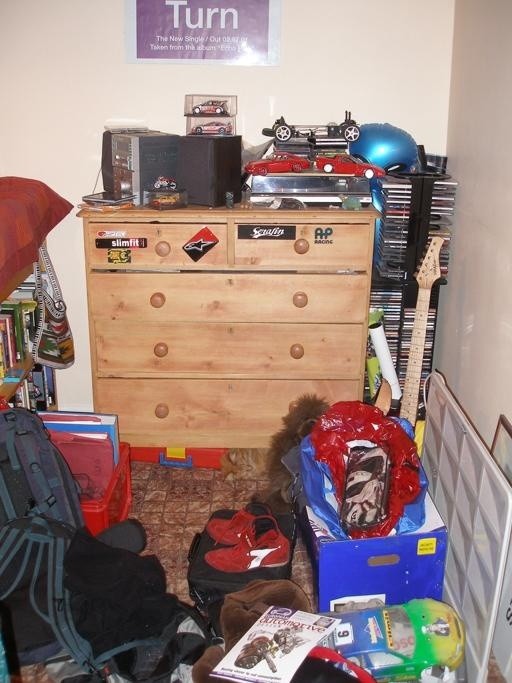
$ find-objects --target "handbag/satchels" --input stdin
[28,245,79,372]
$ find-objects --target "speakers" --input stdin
[101,129,159,192]
[139,135,242,207]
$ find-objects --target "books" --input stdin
[103,122,148,134]
[0,271,58,416]
[207,602,342,683]
[362,171,459,418]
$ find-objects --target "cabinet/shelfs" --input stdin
[76,194,382,448]
[0,243,58,417]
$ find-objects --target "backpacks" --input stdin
[0,514,106,674]
[0,405,85,529]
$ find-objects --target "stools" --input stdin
[44,435,133,536]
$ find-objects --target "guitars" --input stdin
[371,234,452,434]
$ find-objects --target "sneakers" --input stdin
[203,502,291,574]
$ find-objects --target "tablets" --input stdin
[83,192,137,203]
